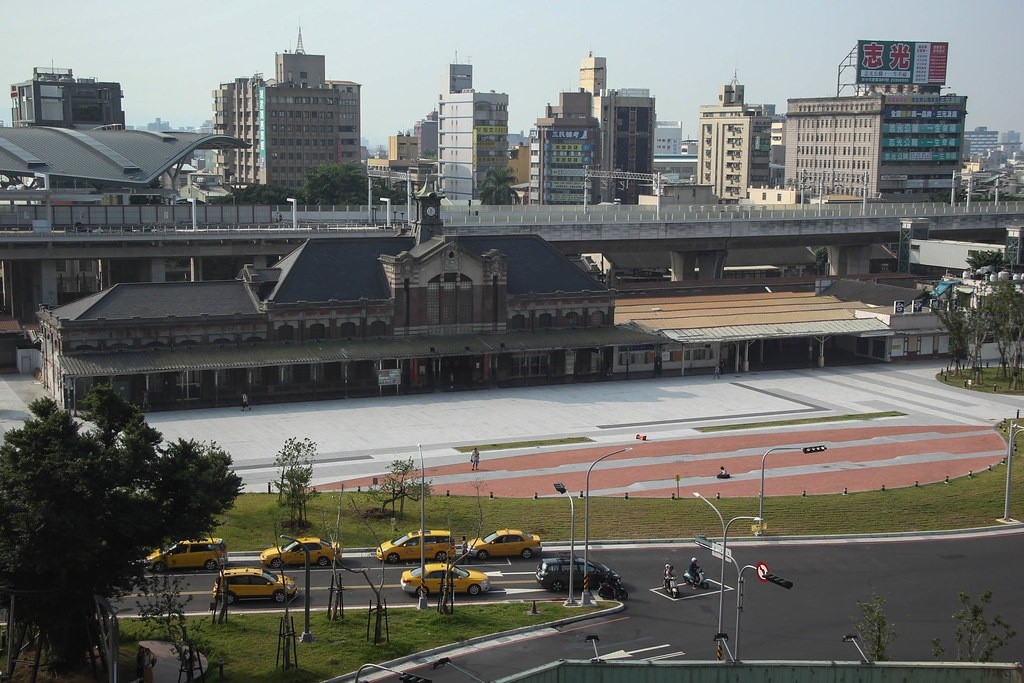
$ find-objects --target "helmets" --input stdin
[668,565,673,570]
[665,564,670,569]
[691,558,697,563]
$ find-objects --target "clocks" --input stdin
[427,206,436,217]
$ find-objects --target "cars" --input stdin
[400,563,491,597]
[466,529,544,561]
[259,535,342,570]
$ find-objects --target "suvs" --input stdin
[212,566,298,606]
[535,551,622,594]
[144,536,229,573]
[375,529,456,564]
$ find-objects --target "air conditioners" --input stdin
[481,137,486,142]
[328,100,333,104]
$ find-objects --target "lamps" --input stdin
[465,346,471,352]
[500,342,505,348]
[430,346,435,353]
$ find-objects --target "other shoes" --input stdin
[692,586,696,590]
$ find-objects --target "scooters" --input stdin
[683,565,709,589]
[661,576,679,599]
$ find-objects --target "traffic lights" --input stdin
[763,572,793,589]
[399,672,432,683]
[804,445,828,454]
[554,483,566,493]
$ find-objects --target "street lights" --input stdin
[94,594,135,683]
[417,442,427,611]
[279,534,313,644]
[694,491,764,661]
[581,447,634,605]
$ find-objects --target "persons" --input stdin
[605,570,619,601]
[142,390,152,410]
[471,448,479,470]
[713,364,720,379]
[688,557,700,590]
[240,392,251,411]
[144,648,154,683]
[136,645,144,683]
[461,536,471,564]
[663,564,681,595]
[720,358,725,374]
[449,371,454,388]
